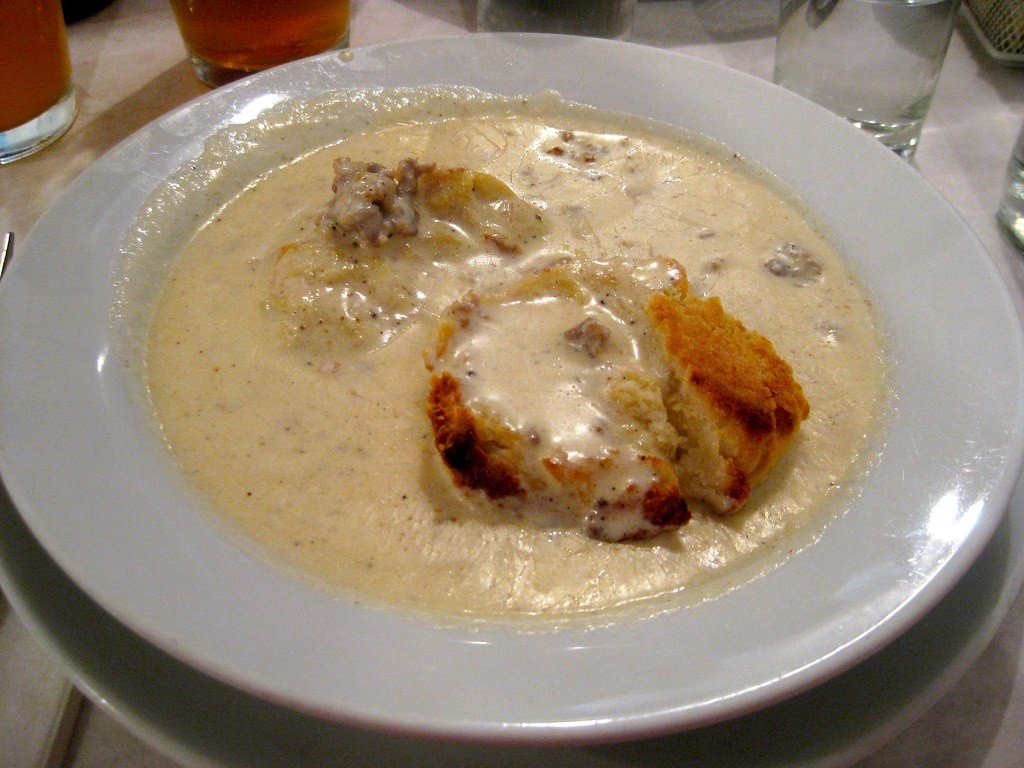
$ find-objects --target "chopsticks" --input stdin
[0,233,82,768]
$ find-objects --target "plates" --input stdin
[1,486,1024,768]
[0,31,1024,746]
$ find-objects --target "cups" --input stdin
[997,122,1024,252]
[773,0,960,161]
[0,0,78,165]
[477,0,633,41]
[170,0,349,89]
